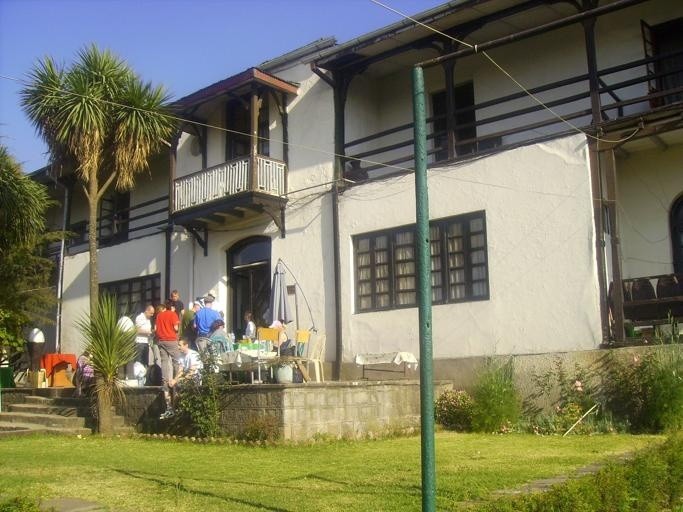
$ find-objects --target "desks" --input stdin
[350,350,417,382]
[38,352,78,389]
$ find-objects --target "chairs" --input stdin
[605,272,682,340]
[192,324,327,385]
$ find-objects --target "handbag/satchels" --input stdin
[146,363,162,386]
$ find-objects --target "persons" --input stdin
[72,289,288,420]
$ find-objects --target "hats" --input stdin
[194,289,216,309]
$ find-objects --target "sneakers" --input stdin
[159,409,175,420]
